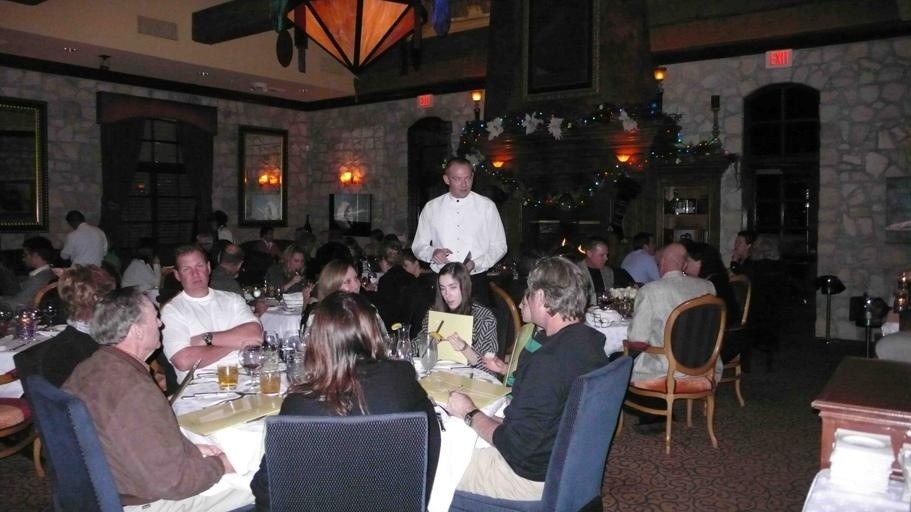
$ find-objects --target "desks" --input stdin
[811,354,911,483]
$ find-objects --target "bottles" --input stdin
[896,271,911,289]
[893,288,909,313]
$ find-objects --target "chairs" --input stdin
[719,273,752,406]
[615,292,726,454]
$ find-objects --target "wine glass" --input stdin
[273,284,284,308]
[618,303,631,322]
[368,273,377,291]
[250,287,263,299]
[600,289,615,311]
[0,298,60,342]
[237,320,438,394]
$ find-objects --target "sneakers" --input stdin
[633,421,679,436]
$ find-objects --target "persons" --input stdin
[563,230,767,431]
[250,291,441,510]
[410,157,508,310]
[40,263,258,512]
[161,245,264,384]
[447,256,608,512]
[406,262,499,378]
[2,210,400,324]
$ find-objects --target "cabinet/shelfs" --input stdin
[651,153,735,256]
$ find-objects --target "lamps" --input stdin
[471,91,482,120]
[655,72,664,112]
[284,0,429,80]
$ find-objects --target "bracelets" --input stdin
[202,332,214,346]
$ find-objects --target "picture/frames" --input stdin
[237,123,288,228]
[520,0,601,105]
[0,94,49,233]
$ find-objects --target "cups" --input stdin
[217,361,239,391]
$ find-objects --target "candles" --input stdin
[711,95,720,108]
